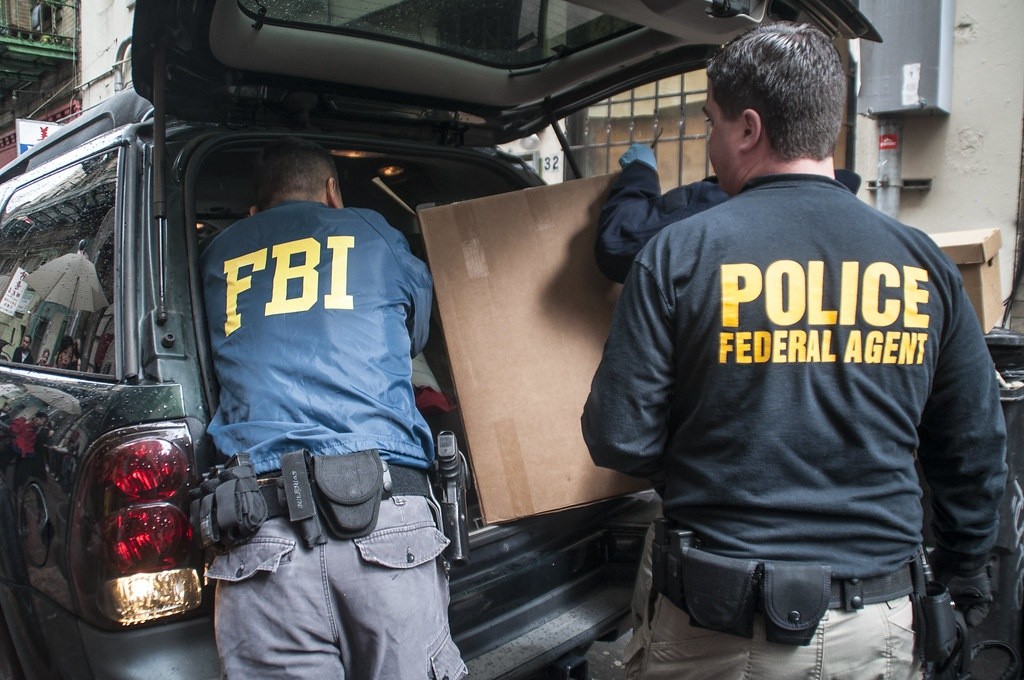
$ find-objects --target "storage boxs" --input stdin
[923,226,1006,333]
[421,168,655,524]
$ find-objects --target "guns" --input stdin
[437,429,472,569]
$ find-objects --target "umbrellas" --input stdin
[24,253,109,331]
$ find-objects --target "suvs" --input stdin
[0,0,883,679]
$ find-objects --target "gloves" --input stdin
[619,143,658,172]
[940,564,990,626]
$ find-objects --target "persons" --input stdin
[200,138,467,680]
[595,143,863,284]
[580,21,1009,680]
[10,334,76,369]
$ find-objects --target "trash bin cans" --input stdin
[984,329,1024,491]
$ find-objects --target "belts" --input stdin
[253,462,433,522]
[659,554,915,610]
